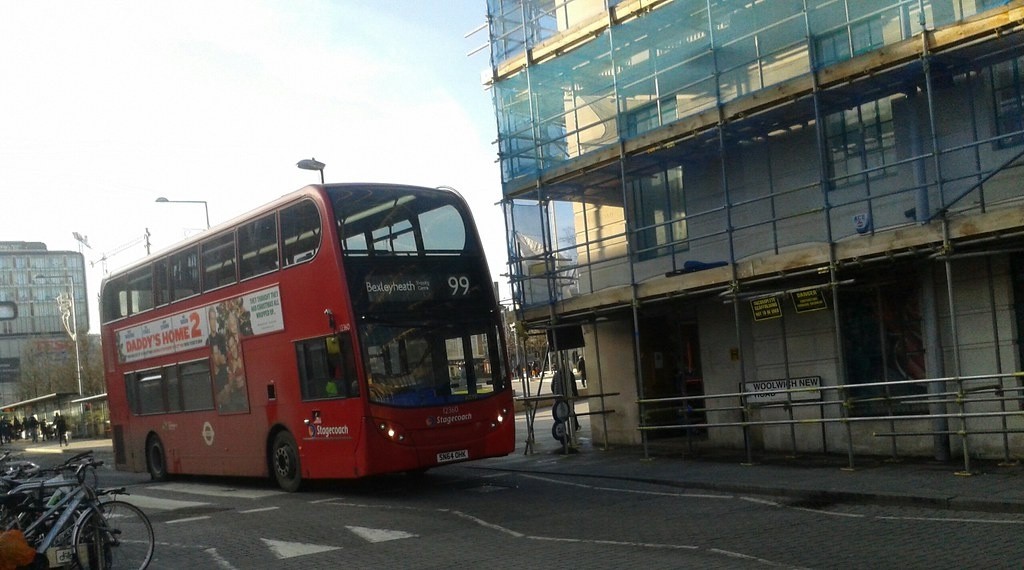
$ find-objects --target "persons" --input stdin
[576,356,586,388]
[550,365,582,433]
[203,298,254,415]
[56,415,69,447]
[0,413,60,444]
[514,361,538,382]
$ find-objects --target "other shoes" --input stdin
[576,426,582,430]
[58,444,63,447]
[65,443,70,446]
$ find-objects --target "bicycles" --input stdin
[0,449,155,570]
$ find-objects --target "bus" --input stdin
[98,184,515,493]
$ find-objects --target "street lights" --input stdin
[155,197,211,231]
[32,272,84,414]
[296,158,326,183]
